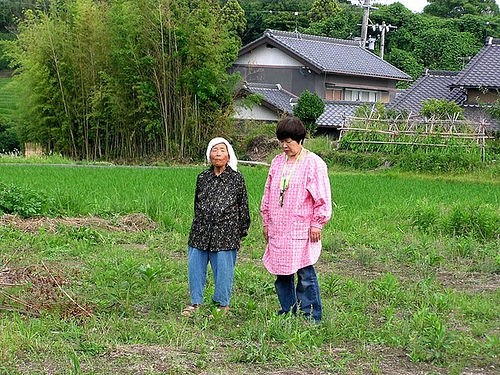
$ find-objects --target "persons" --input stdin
[182,136,251,319]
[260,117,332,328]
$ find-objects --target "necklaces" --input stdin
[283,151,299,188]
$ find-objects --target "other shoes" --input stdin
[181,304,201,316]
[208,306,232,319]
